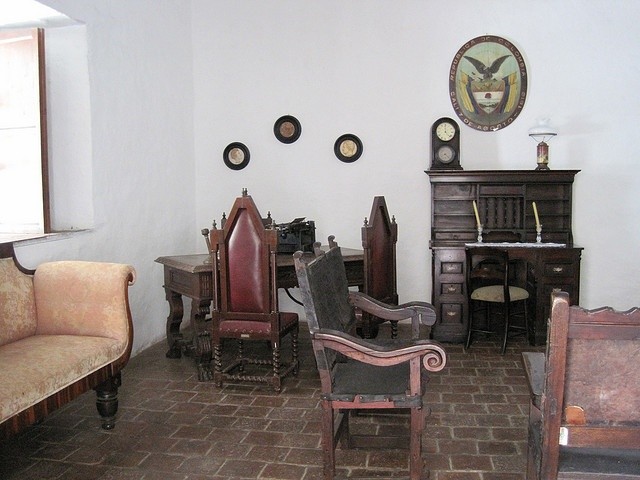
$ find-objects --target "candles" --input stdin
[531,200,540,229]
[472,200,482,229]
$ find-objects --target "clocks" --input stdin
[274,116,301,143]
[334,134,363,162]
[222,142,250,170]
[429,117,463,170]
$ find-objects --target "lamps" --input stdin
[528,126,558,170]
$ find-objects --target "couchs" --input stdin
[0,243,136,442]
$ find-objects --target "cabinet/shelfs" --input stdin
[481,183,523,242]
[523,182,569,242]
[431,249,468,342]
[534,248,581,346]
[433,183,481,244]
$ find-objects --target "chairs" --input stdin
[212,186,298,394]
[361,197,398,339]
[520,287,640,479]
[293,235,447,480]
[466,247,529,355]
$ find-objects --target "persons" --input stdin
[342,140,356,157]
[231,150,243,164]
[281,122,295,138]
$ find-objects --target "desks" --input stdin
[153,247,365,382]
